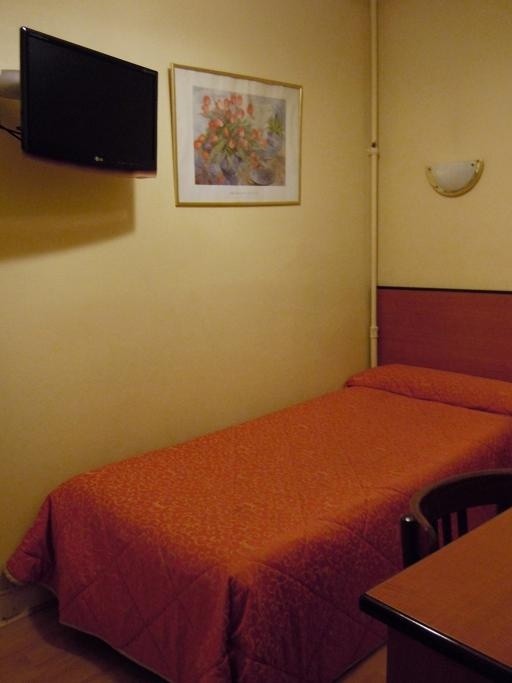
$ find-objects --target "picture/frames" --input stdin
[171,62,303,206]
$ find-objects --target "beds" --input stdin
[5,285,512,683]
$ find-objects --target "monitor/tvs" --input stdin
[20,26,158,171]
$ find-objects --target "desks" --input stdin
[357,508,512,683]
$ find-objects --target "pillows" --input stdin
[344,362,512,414]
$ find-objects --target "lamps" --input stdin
[425,162,485,197]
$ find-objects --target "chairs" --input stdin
[400,469,512,569]
